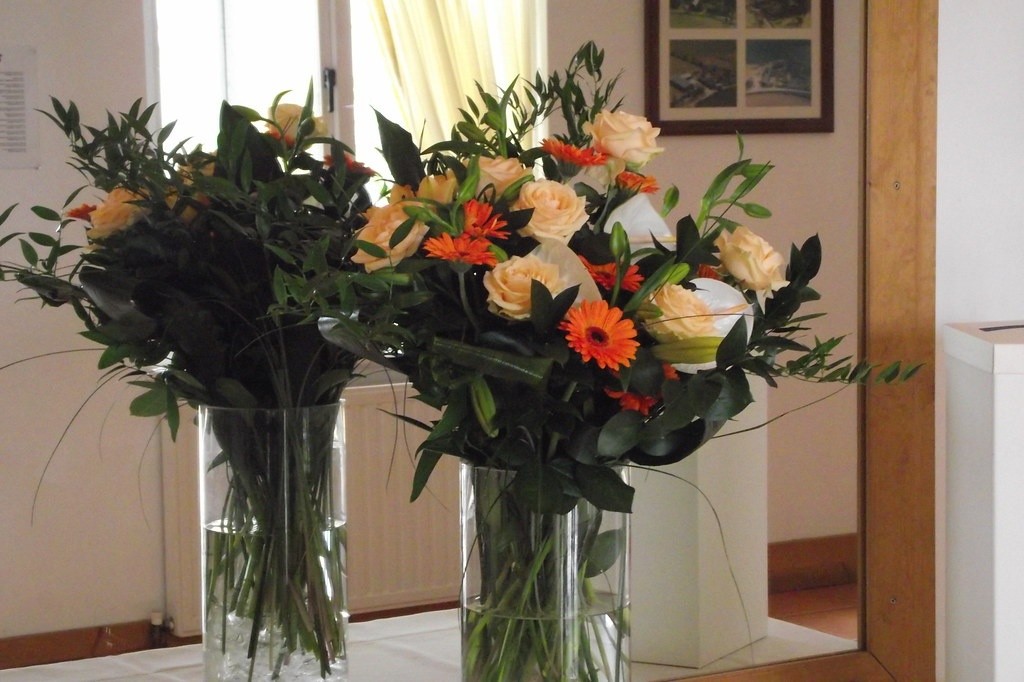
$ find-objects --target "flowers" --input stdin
[0,94,390,441]
[281,42,929,512]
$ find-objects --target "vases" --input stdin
[198,399,347,682]
[461,459,631,682]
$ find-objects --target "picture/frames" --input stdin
[644,0,835,136]
